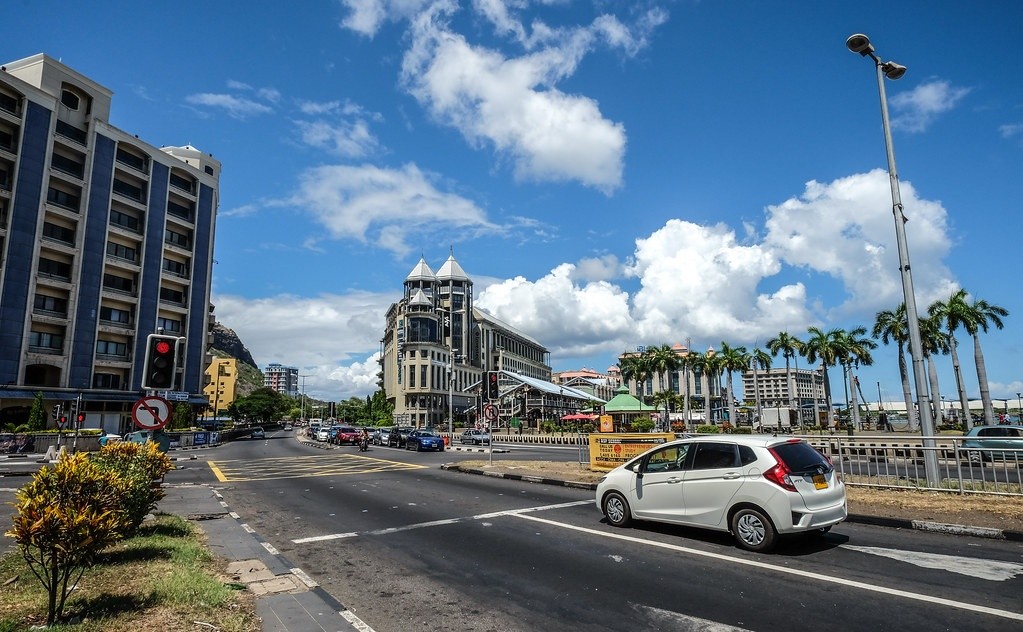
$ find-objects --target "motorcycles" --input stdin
[360,436,369,451]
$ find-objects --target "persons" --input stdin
[390,424,399,435]
[735,415,752,427]
[1018,411,1023,426]
[974,413,984,424]
[360,427,369,448]
[866,415,872,430]
[518,422,523,435]
[999,412,1010,425]
[98,432,108,447]
[723,420,728,433]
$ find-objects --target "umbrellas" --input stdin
[561,413,595,420]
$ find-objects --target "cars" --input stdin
[962,424,1023,463]
[595,433,849,552]
[251,427,265,439]
[324,419,335,426]
[406,429,444,452]
[277,420,308,431]
[307,427,411,448]
[460,429,491,446]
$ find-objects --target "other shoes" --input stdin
[358,448,360,452]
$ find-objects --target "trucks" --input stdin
[309,419,322,427]
[752,408,798,433]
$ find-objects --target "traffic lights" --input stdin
[71,397,79,416]
[79,412,86,422]
[52,405,60,420]
[142,332,176,390]
[59,416,67,422]
[488,371,499,400]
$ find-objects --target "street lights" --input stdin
[213,362,229,431]
[291,373,318,433]
[846,32,942,488]
[436,307,466,446]
[560,387,563,436]
[844,366,859,442]
[638,380,643,431]
[774,379,782,430]
[1017,392,1022,413]
[940,395,947,419]
